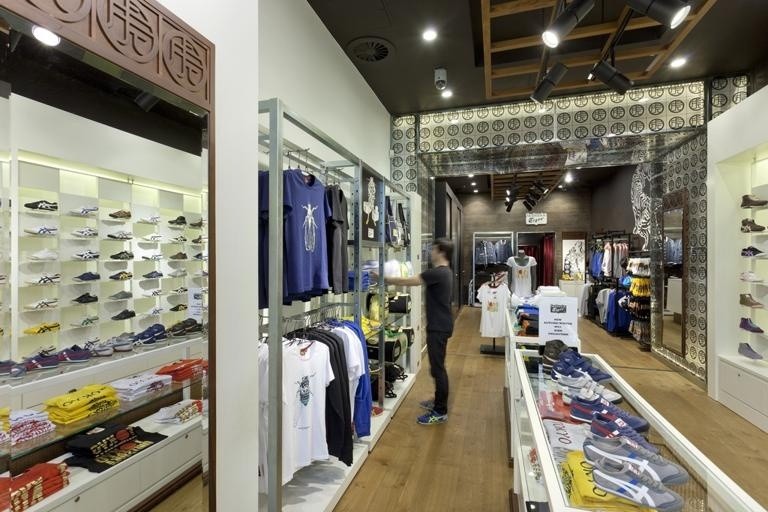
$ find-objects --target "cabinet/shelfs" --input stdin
[707,84,768,434]
[502,304,768,512]
[259,99,421,512]
[0,93,210,512]
[473,231,514,307]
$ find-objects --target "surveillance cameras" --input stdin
[432,69,448,90]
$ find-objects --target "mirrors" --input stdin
[661,187,685,358]
[0,0,217,512]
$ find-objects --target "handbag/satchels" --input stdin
[368,194,415,402]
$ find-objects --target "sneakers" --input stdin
[420,399,435,410]
[0,201,207,376]
[738,194,767,359]
[543,340,689,512]
[417,411,448,425]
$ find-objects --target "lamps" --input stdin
[504,188,519,212]
[523,182,549,211]
[531,0,596,103]
[590,0,691,95]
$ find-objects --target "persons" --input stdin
[506,250,537,299]
[370,238,454,424]
